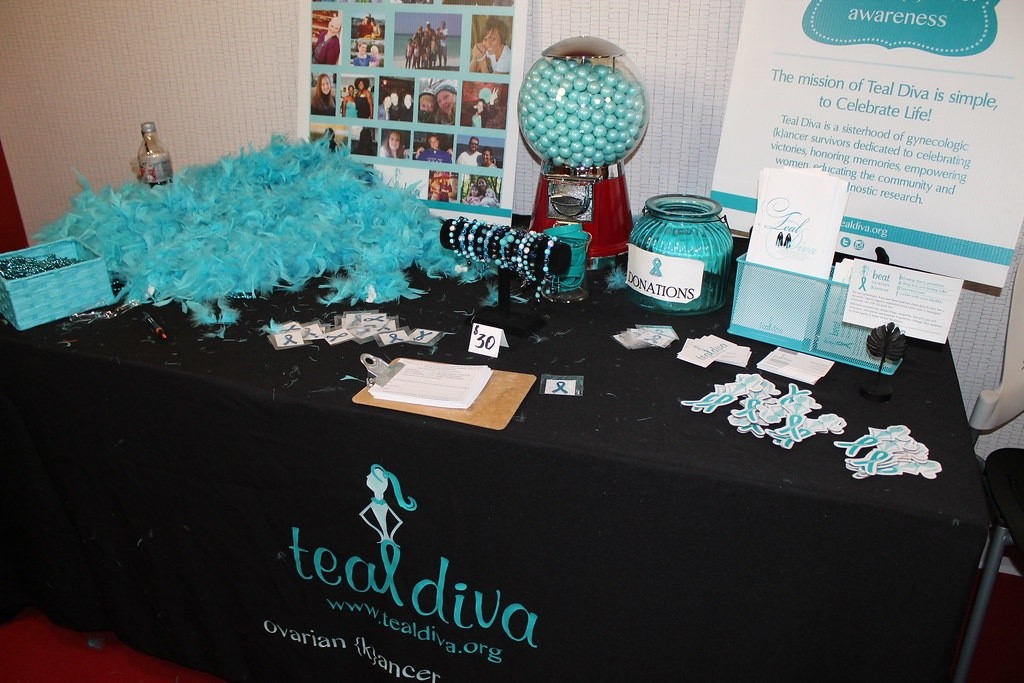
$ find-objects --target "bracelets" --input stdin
[449,215,562,303]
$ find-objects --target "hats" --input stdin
[365,13,372,19]
[419,89,435,97]
[434,83,457,95]
[426,22,430,25]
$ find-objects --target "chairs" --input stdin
[952,449,1024,682]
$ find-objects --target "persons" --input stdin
[310,0,513,211]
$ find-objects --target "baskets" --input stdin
[727,253,903,375]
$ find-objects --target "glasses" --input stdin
[470,143,478,146]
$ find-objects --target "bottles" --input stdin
[137,122,173,188]
[630,193,734,315]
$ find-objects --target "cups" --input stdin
[558,232,592,286]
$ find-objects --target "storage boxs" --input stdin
[727,252,921,375]
[0,236,120,332]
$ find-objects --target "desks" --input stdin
[0,213,974,682]
